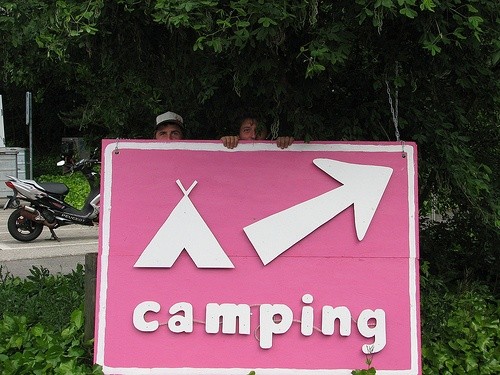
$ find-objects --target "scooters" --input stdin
[3,147,102,242]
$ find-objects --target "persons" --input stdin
[220,98,296,149]
[155,112,186,139]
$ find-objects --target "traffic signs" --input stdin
[94,139,421,375]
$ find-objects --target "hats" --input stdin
[153,110,185,130]
